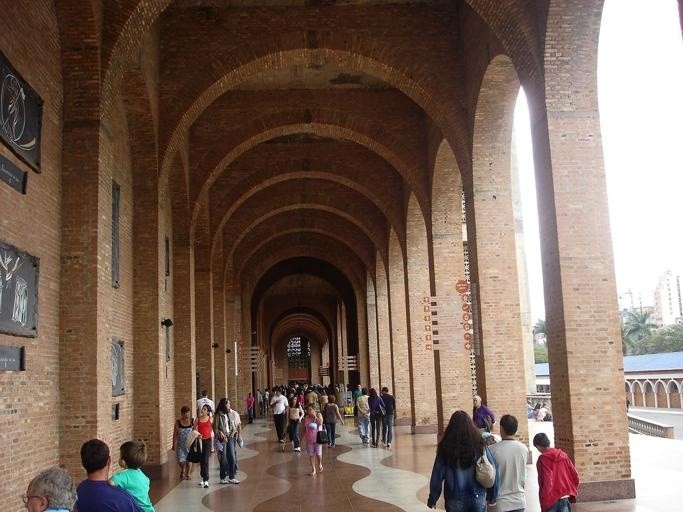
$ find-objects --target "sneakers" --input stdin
[180,472,240,488]
[294,447,301,452]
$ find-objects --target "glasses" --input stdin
[21,494,41,504]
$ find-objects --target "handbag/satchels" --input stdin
[186,452,202,463]
[482,414,493,433]
[476,456,496,488]
[307,422,317,430]
[377,404,386,416]
[214,440,223,452]
[316,431,329,444]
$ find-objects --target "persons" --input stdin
[425,410,498,511]
[193,404,214,488]
[532,434,580,511]
[527,400,534,418]
[195,388,215,414]
[212,397,240,484]
[171,406,196,481]
[75,437,144,512]
[471,394,496,431]
[247,382,392,453]
[20,466,78,512]
[224,399,243,478]
[481,431,501,446]
[299,403,324,476]
[533,403,541,419]
[107,440,154,512]
[626,397,630,413]
[486,414,529,512]
[536,402,551,421]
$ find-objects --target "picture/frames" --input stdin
[111,339,126,397]
[0,238,42,338]
[0,45,46,176]
[112,178,121,288]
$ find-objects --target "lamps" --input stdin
[226,348,232,354]
[160,316,175,330]
[211,343,219,350]
